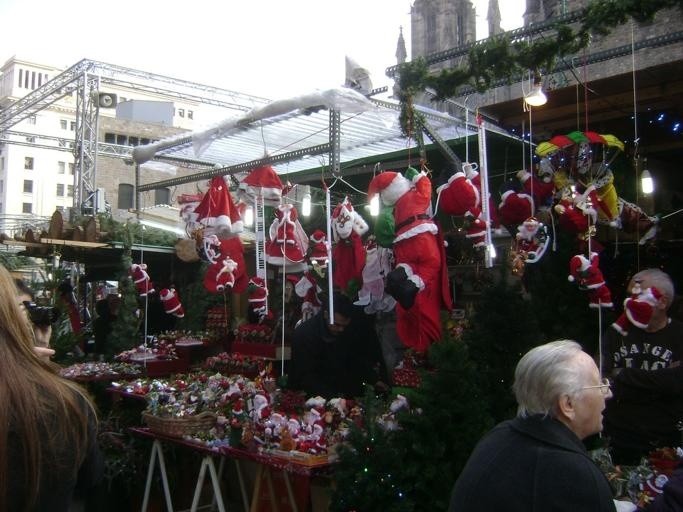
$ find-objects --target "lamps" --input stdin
[243,204,253,227]
[300,193,313,217]
[522,72,548,106]
[640,168,654,194]
[370,192,379,215]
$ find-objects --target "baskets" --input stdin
[143,411,217,439]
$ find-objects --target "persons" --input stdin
[595,175,620,229]
[292,293,363,397]
[14,280,53,362]
[310,227,328,265]
[160,286,185,318]
[572,250,615,313]
[258,203,309,275]
[625,462,680,512]
[128,262,157,297]
[191,165,249,295]
[434,162,491,252]
[95,294,103,301]
[496,184,533,226]
[357,236,396,311]
[249,276,271,318]
[513,219,554,266]
[109,325,370,466]
[205,306,231,326]
[520,168,553,206]
[597,266,675,439]
[1,264,107,510]
[611,280,661,336]
[286,269,324,324]
[330,196,367,288]
[449,337,617,511]
[375,169,452,353]
[393,347,431,385]
[235,156,283,209]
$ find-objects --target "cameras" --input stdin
[23,301,60,327]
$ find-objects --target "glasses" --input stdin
[569,377,611,394]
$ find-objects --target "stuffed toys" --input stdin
[378,395,423,432]
[534,131,624,237]
[625,462,678,509]
[60,360,107,387]
[590,443,617,496]
[643,446,682,462]
[618,447,624,503]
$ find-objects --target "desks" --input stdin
[77,377,112,382]
[106,386,145,402]
[134,359,179,365]
[127,426,335,511]
[175,340,203,347]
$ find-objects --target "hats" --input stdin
[189,162,243,237]
[239,166,282,207]
[260,240,308,266]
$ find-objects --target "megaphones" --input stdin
[91,91,117,108]
